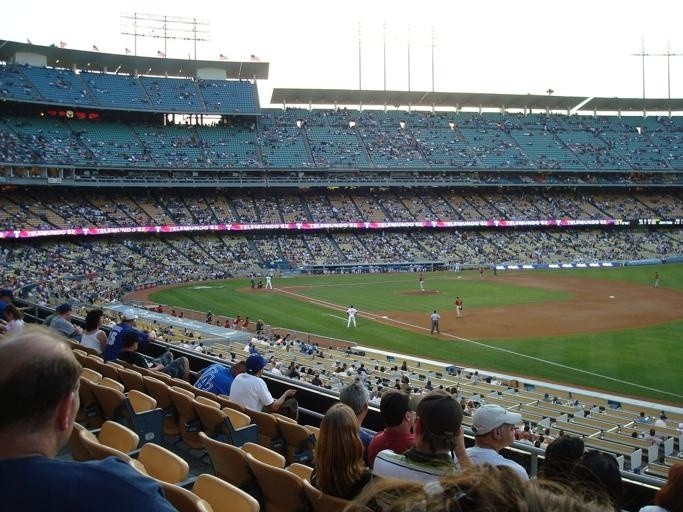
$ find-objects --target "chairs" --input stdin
[0,59,682,276]
[271,337,682,512]
[1,276,270,511]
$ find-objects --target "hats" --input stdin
[245,355,266,374]
[119,332,137,348]
[470,403,521,436]
[123,309,138,320]
[407,389,462,437]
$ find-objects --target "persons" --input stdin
[428,309,441,334]
[0,324,183,511]
[0,58,683,312]
[344,304,358,327]
[41,297,682,512]
[453,295,462,319]
